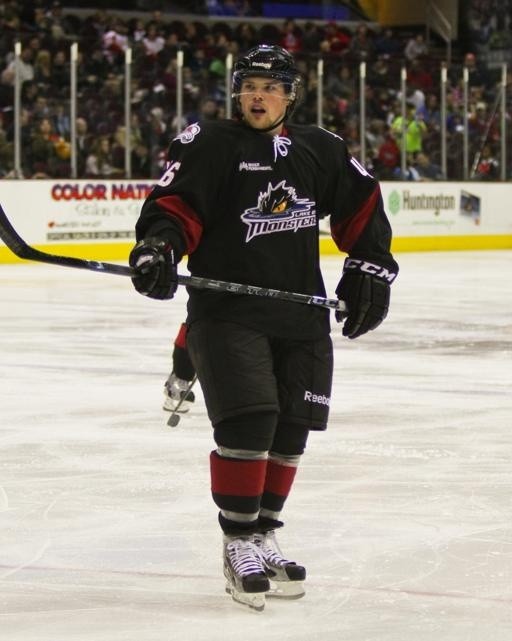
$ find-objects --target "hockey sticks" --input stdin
[167,376,197,427]
[0,207,351,315]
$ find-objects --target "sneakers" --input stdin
[164,372,194,402]
[224,522,306,592]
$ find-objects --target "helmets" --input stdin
[231,43,301,100]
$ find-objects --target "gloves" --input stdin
[129,235,178,300]
[336,252,398,338]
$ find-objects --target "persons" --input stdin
[163,324,195,403]
[129,45,399,610]
[1,1,512,182]
